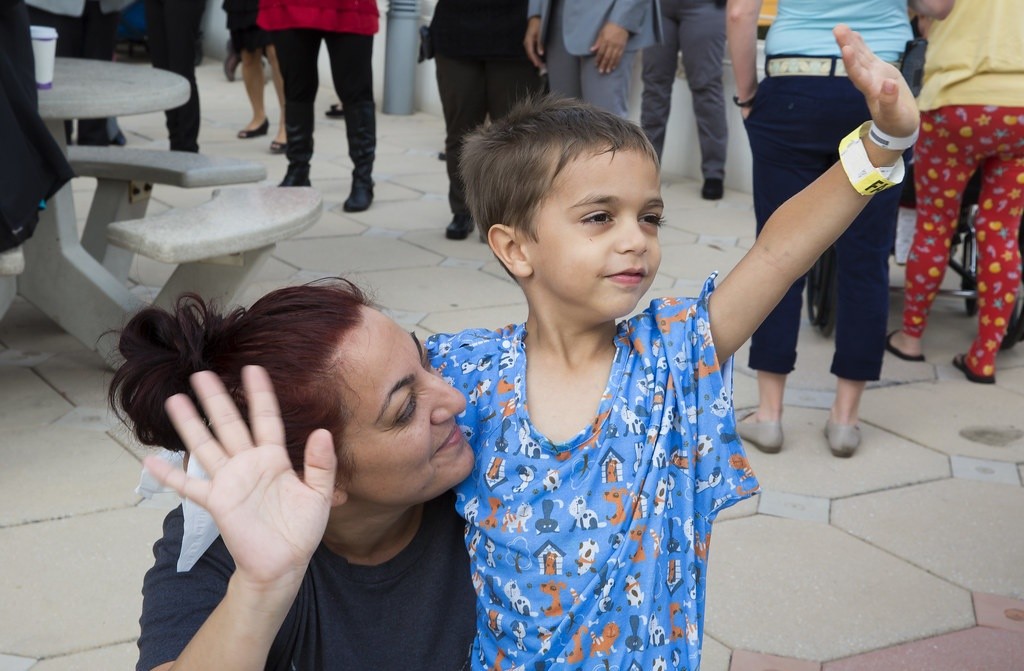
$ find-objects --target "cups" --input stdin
[29,26,59,90]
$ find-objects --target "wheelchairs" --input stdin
[804,32,1024,352]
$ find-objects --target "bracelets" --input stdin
[869,123,919,150]
[733,94,755,107]
[837,121,906,195]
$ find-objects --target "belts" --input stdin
[765,57,903,78]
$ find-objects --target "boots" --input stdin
[343,99,375,212]
[280,102,315,188]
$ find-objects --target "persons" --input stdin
[726,0,955,457]
[107,279,477,671]
[418,0,727,240]
[424,26,920,671]
[0,0,379,245]
[883,0,1024,384]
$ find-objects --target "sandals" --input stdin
[269,139,286,152]
[237,115,268,138]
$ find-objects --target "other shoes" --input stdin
[224,40,239,82]
[444,211,475,240]
[823,420,861,458]
[325,102,342,116]
[700,179,724,199]
[733,411,784,453]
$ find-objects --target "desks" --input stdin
[0,57,277,373]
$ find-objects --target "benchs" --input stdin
[101,184,326,263]
[62,143,270,190]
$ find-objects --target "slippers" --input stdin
[887,329,927,362]
[953,352,995,383]
